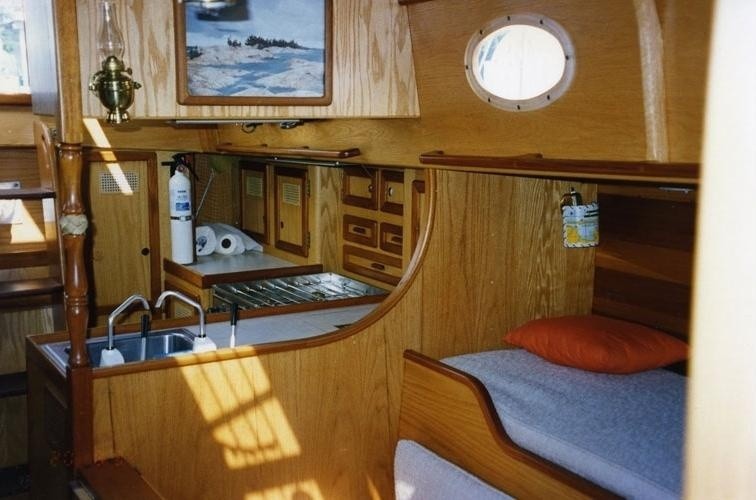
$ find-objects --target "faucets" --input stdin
[154,289,206,342]
[107,293,150,349]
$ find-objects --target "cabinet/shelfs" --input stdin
[164,272,212,321]
[80,147,161,319]
[238,159,311,257]
[340,166,403,286]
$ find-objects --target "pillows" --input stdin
[501,314,687,375]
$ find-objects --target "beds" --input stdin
[395,351,685,499]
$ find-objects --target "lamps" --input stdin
[90,1,142,127]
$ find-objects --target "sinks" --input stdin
[144,328,198,359]
[44,333,160,377]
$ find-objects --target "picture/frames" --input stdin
[173,0,333,104]
